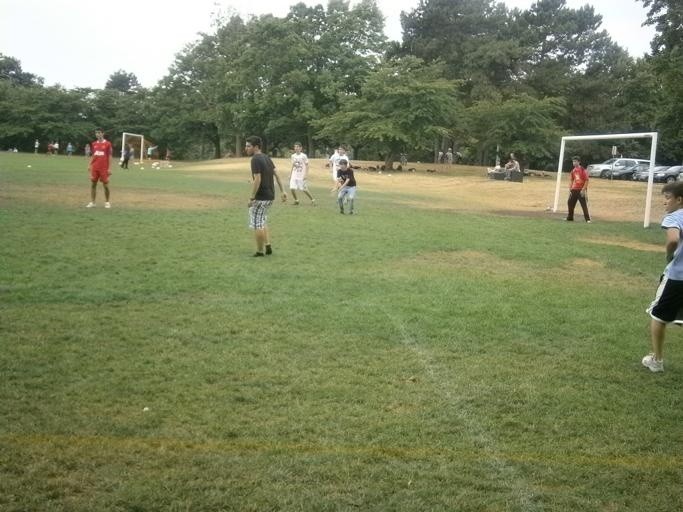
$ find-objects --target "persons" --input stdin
[245,134,288,257]
[8,138,175,169]
[487,152,520,181]
[331,158,358,214]
[85,127,113,209]
[437,146,464,174]
[563,155,592,224]
[329,145,350,191]
[640,180,683,372]
[288,140,317,206]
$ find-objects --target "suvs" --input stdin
[587,157,652,180]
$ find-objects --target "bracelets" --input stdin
[582,188,585,191]
[250,197,256,201]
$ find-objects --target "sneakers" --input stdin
[266,244,272,255]
[86,202,96,207]
[253,251,263,257]
[340,208,344,213]
[642,353,664,374]
[104,202,110,208]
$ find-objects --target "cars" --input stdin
[606,164,683,183]
